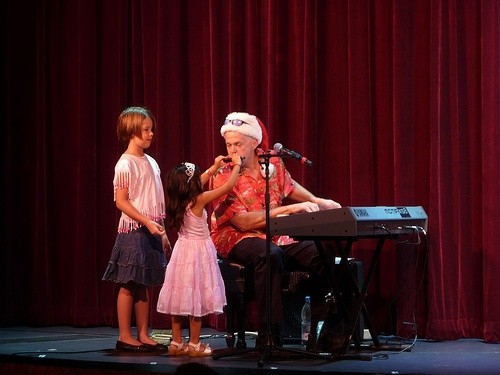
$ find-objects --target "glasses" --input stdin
[224,119,251,126]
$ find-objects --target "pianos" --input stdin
[270,205,430,237]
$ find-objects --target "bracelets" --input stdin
[232,163,241,167]
[204,169,213,177]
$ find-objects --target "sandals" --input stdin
[188,342,213,356]
[169,340,188,354]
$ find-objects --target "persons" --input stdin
[156,154,242,356]
[209,111,358,353]
[101,107,172,352]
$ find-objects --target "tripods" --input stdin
[211,154,333,368]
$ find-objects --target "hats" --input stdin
[220,112,268,154]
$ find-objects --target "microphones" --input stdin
[274,142,312,165]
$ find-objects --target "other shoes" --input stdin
[143,342,168,352]
[116,340,151,353]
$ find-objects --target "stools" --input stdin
[217,260,311,350]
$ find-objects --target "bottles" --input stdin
[301,296,312,344]
